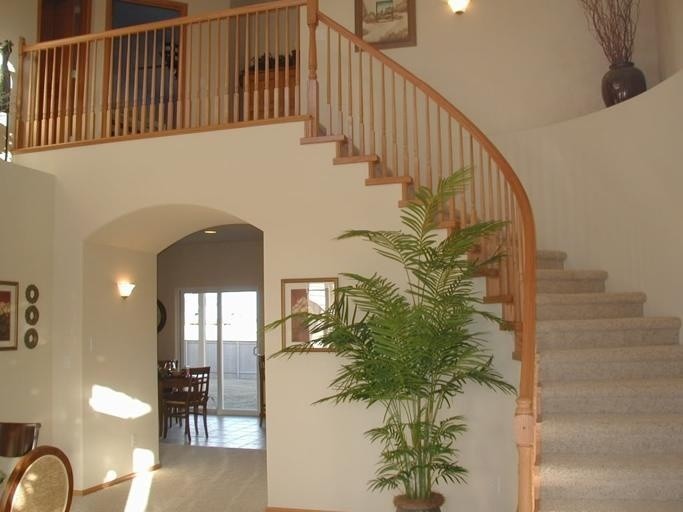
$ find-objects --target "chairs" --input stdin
[0,423,78,512]
[157,359,211,441]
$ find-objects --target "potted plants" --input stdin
[239,48,298,122]
[576,0,648,108]
[260,166,519,512]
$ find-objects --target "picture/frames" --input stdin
[279,277,340,356]
[0,280,20,351]
[355,0,416,51]
[157,299,167,334]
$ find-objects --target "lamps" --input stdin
[117,283,136,299]
